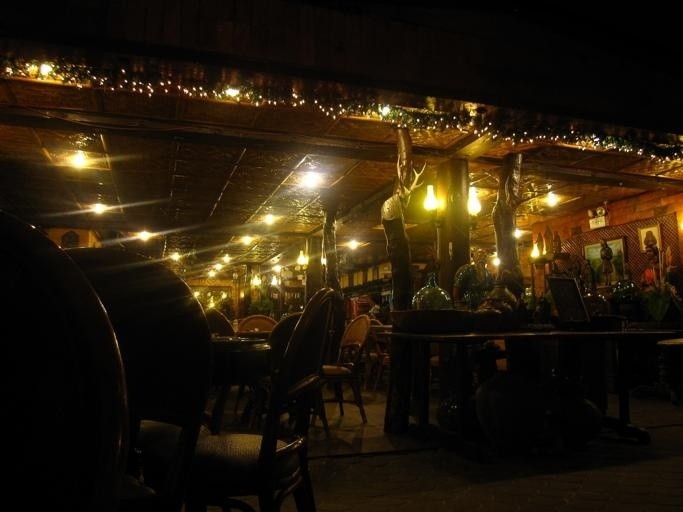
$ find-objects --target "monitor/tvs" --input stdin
[547,274,590,321]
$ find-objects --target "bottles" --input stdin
[233,318,238,332]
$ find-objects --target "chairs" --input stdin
[198,288,375,512]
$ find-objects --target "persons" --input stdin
[452,247,494,308]
[545,252,583,294]
[644,245,659,276]
[61,229,81,247]
[600,237,615,285]
